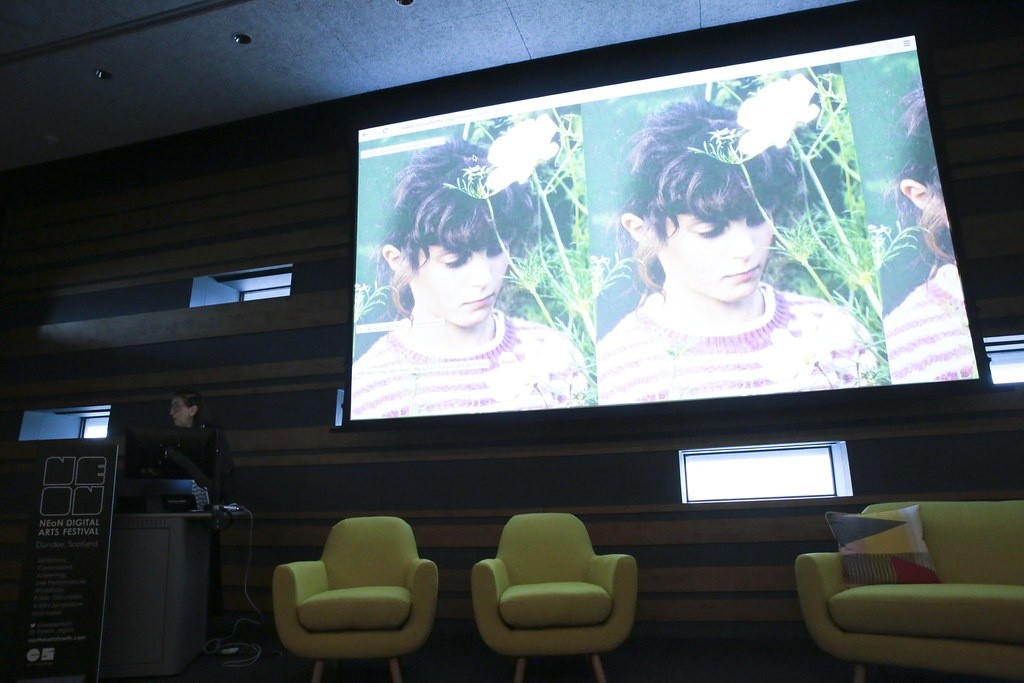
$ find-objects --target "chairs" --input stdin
[470,512,639,683]
[270,514,440,683]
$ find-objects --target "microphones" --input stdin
[204,504,245,512]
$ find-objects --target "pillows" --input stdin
[824,505,943,589]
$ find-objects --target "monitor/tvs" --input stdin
[123,425,220,490]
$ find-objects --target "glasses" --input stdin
[167,402,186,413]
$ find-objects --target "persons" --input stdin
[347,139,589,419]
[880,88,986,386]
[150,388,216,496]
[589,99,878,402]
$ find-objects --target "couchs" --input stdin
[794,502,1024,683]
